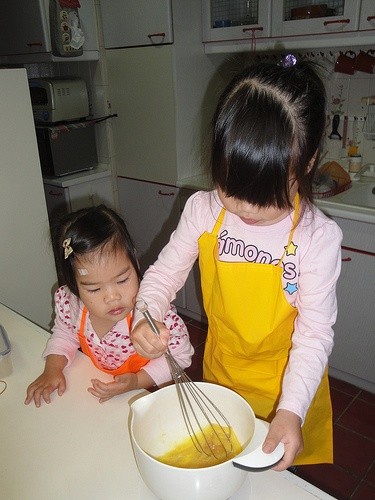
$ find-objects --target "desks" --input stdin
[0,300,340,500]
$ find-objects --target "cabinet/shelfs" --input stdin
[0,0,375,392]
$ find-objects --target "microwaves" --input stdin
[28,75,90,124]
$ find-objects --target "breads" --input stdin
[319,161,351,187]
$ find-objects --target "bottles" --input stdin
[348,154,361,173]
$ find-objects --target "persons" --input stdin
[23,207,193,407]
[129,54,343,477]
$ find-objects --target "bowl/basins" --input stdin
[215,20,230,27]
[290,4,327,21]
[128,380,285,500]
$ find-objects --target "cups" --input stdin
[336,50,356,75]
[354,49,375,74]
[0,324,11,356]
[314,53,335,80]
